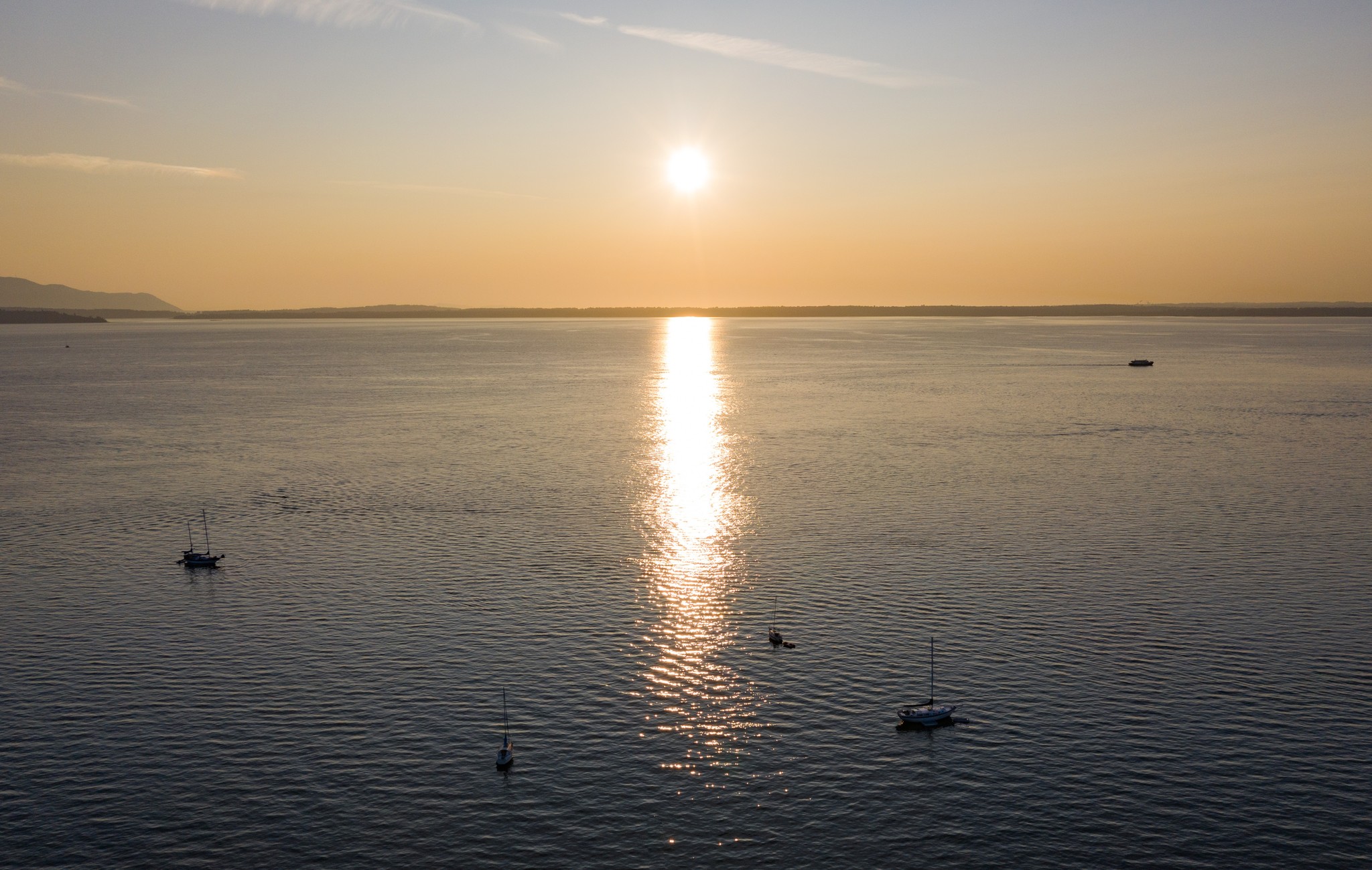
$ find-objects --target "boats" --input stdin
[783,642,795,648]
[1128,358,1154,366]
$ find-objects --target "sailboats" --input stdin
[768,595,783,644]
[177,508,224,569]
[496,686,514,766]
[895,635,964,726]
